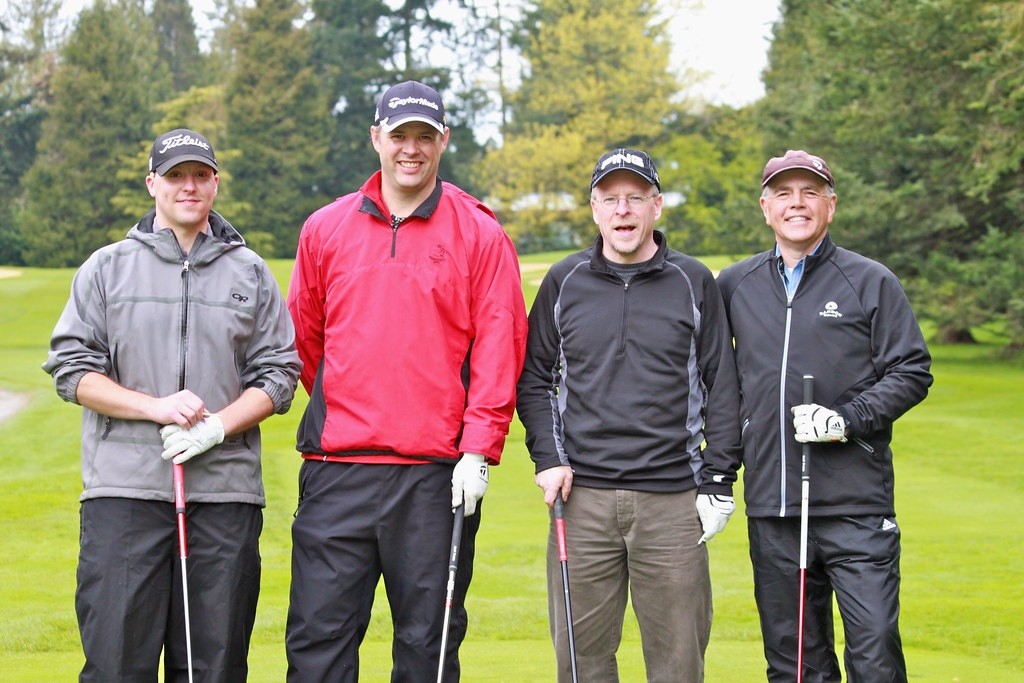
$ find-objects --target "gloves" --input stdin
[161,412,223,466]
[450,451,491,514]
[792,404,848,443]
[694,493,736,545]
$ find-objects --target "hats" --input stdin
[589,149,662,193]
[149,128,218,175]
[373,82,446,134]
[762,150,836,187]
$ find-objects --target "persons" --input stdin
[40,129,298,683]
[282,82,530,683]
[716,151,934,683]
[512,149,742,683]
